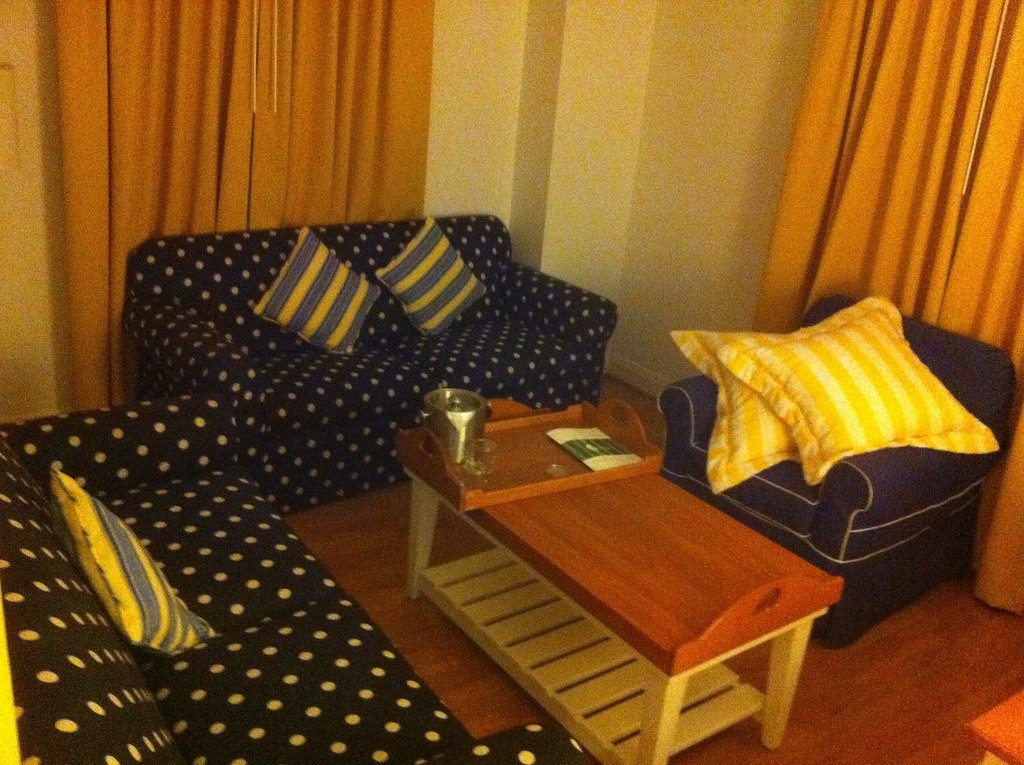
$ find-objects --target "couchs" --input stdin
[0,400,590,765]
[126,216,619,514]
[658,294,1020,647]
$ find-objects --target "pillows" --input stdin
[374,214,487,336]
[671,296,906,498]
[716,296,999,487]
[251,224,384,356]
[53,471,218,656]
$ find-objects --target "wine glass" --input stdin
[463,439,497,487]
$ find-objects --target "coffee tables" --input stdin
[402,425,844,765]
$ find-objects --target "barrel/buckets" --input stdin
[416,388,492,463]
